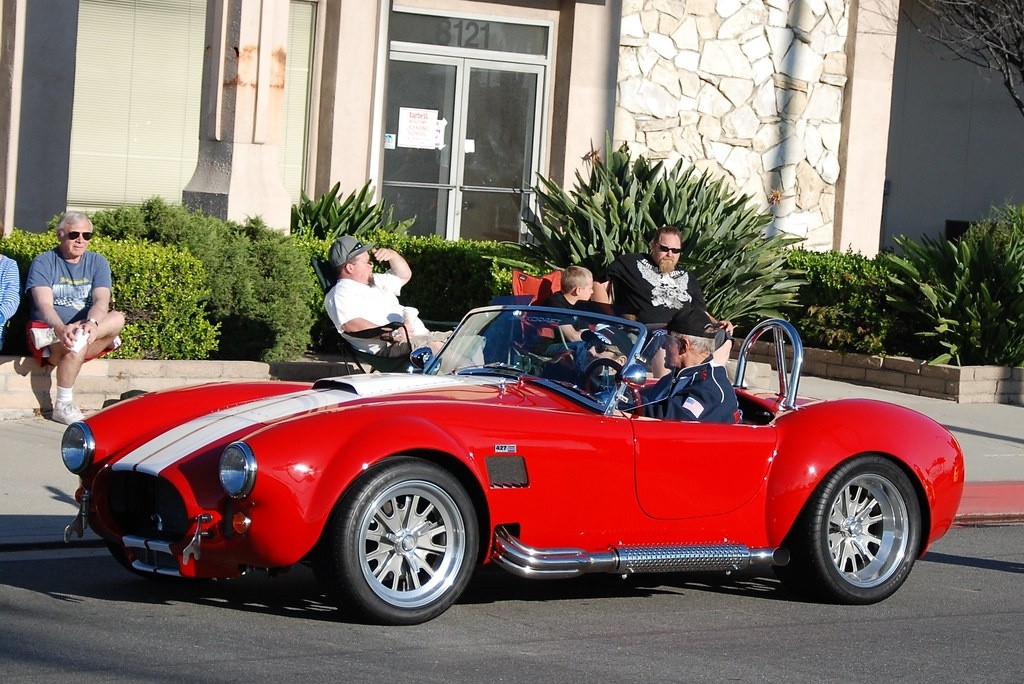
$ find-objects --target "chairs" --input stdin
[732,388,778,427]
[309,256,415,375]
[506,266,577,370]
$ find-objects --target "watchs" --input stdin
[83,319,98,327]
[391,330,398,337]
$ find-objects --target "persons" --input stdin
[590,226,734,379]
[529,266,743,424]
[0,221,20,350]
[324,237,486,374]
[26,211,125,425]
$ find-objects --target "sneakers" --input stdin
[51,399,85,426]
[30,325,66,351]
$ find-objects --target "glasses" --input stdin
[64,231,94,241]
[583,340,623,357]
[653,240,681,254]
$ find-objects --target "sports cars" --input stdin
[61,307,967,629]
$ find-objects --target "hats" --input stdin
[666,305,716,339]
[580,326,634,360]
[328,236,375,270]
